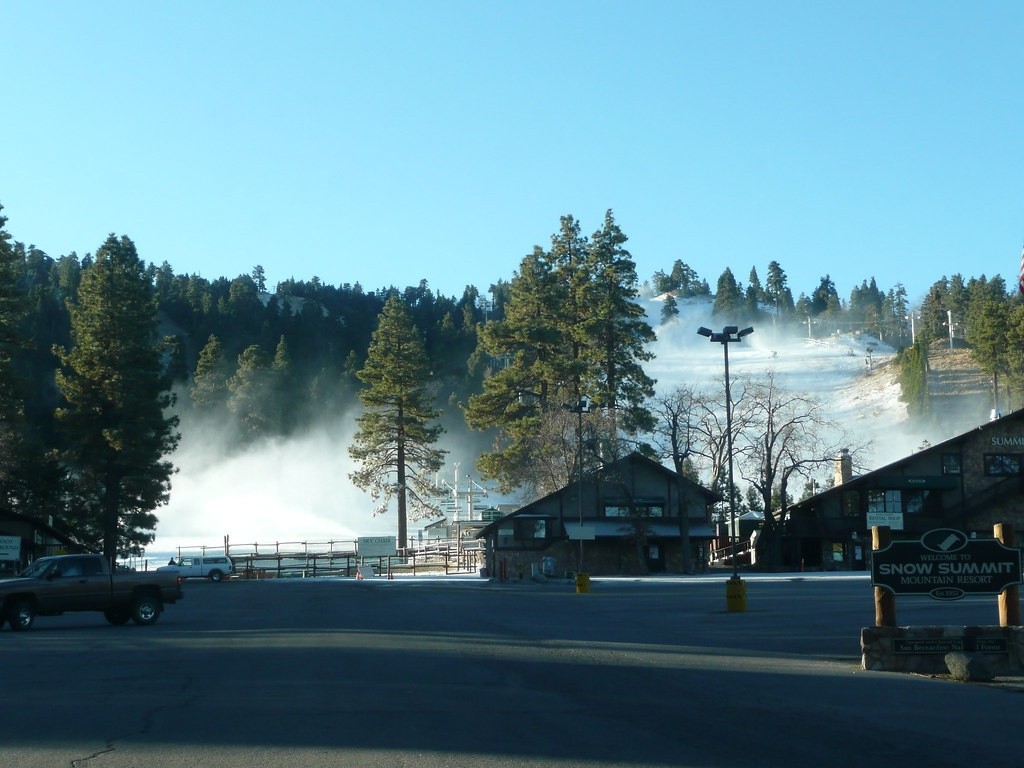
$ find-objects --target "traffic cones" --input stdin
[390,572,394,579]
[356,572,361,580]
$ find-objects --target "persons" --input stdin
[168,557,176,565]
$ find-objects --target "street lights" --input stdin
[697,324,755,613]
[561,400,598,595]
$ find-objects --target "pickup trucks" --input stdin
[156,554,234,582]
[0,553,186,631]
